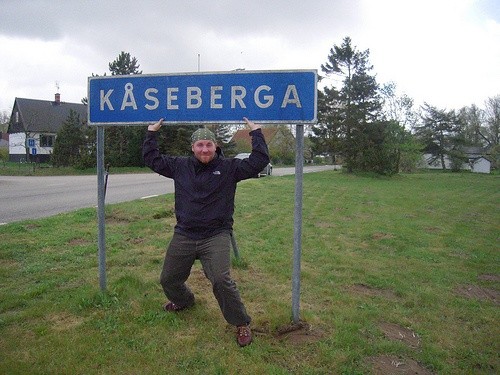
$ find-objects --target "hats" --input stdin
[191,129,216,145]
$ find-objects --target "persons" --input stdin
[138,114,271,347]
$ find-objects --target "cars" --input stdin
[234,151,274,178]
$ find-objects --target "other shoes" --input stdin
[237,323,252,346]
[165,302,182,312]
